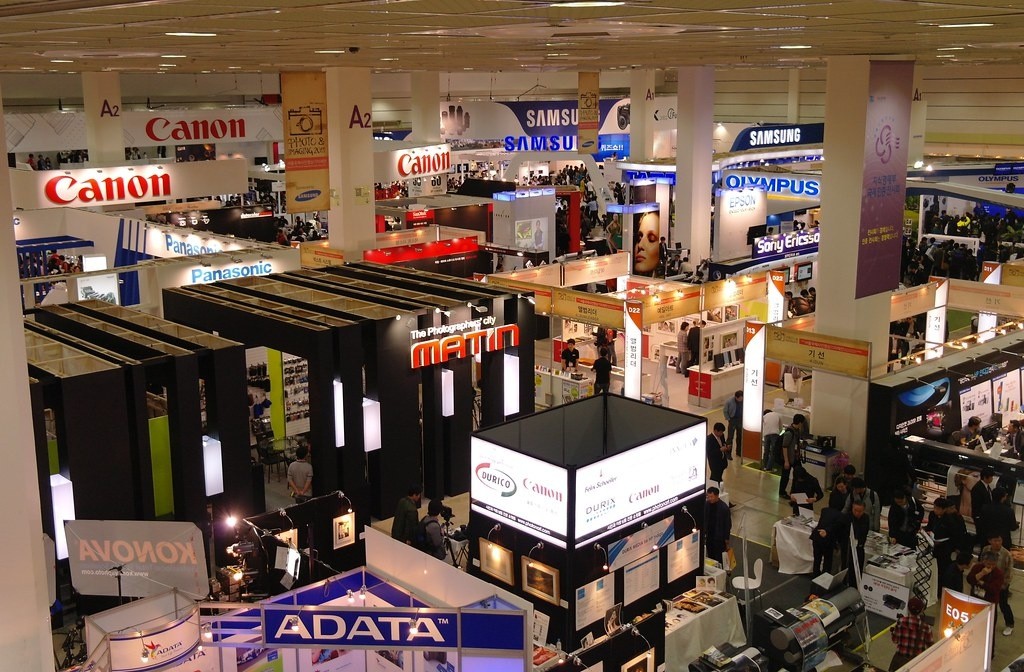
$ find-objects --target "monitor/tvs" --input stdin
[710,347,745,373]
[980,422,998,446]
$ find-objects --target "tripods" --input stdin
[443,517,458,568]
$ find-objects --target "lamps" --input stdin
[593,505,697,570]
[395,292,537,327]
[65,164,162,175]
[198,253,272,268]
[377,241,452,256]
[486,524,543,567]
[913,337,1024,393]
[631,627,651,658]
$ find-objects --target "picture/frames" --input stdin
[656,305,740,365]
[521,556,561,607]
[478,538,514,586]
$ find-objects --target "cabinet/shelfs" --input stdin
[258,435,302,466]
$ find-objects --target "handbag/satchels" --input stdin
[721,546,736,570]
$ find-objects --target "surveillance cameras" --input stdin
[349,47,361,54]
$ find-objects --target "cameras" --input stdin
[440,505,452,518]
[617,103,631,130]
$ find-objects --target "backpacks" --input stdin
[772,428,794,456]
[414,516,441,555]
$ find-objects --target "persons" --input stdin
[522,164,602,256]
[675,320,706,378]
[288,446,313,503]
[561,327,618,394]
[705,486,733,576]
[767,220,819,236]
[659,237,693,276]
[889,312,949,364]
[759,408,1024,672]
[723,390,743,460]
[785,286,817,317]
[386,218,401,231]
[947,468,980,518]
[126,148,140,160]
[608,180,626,206]
[391,484,446,560]
[900,202,1024,287]
[447,170,500,192]
[215,191,322,246]
[612,152,617,159]
[707,422,737,508]
[605,214,621,254]
[27,150,88,171]
[17,249,83,291]
[633,211,660,277]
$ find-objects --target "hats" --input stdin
[908,598,924,610]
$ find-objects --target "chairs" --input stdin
[733,558,763,608]
[261,450,288,484]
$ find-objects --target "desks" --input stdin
[534,335,744,407]
[664,589,746,672]
[769,446,938,608]
[446,526,469,564]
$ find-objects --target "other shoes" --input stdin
[780,493,790,500]
[767,468,778,473]
[1002,626,1014,636]
[764,467,767,470]
[728,456,732,460]
[729,503,736,509]
